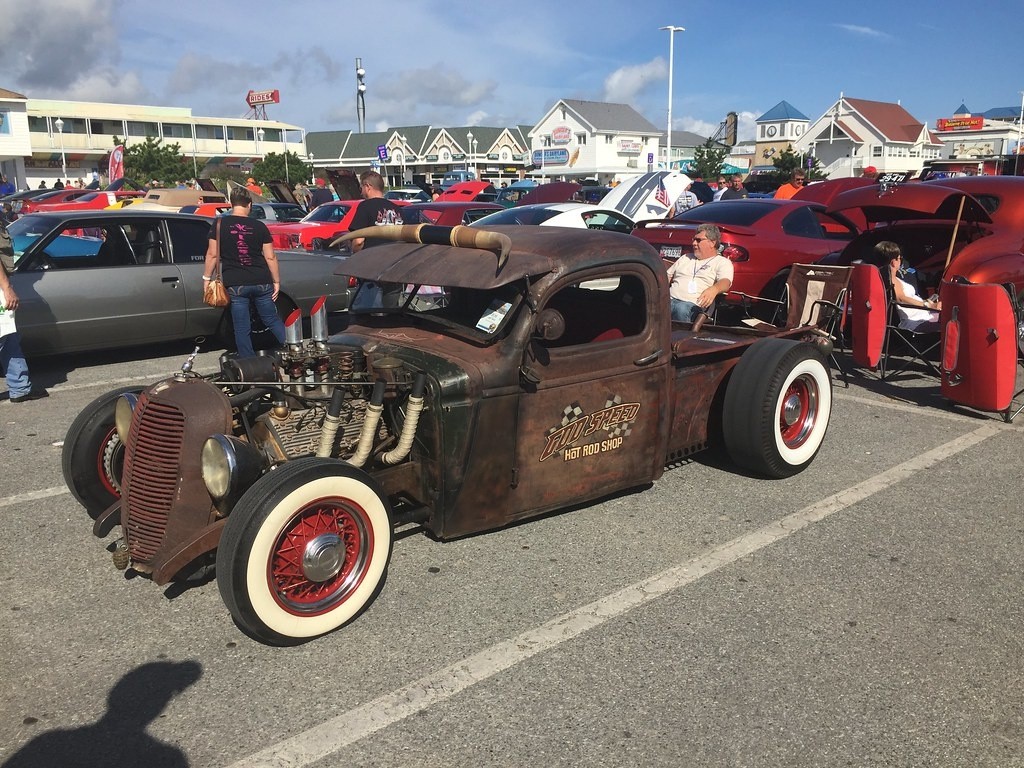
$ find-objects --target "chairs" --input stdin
[98,223,159,265]
[660,258,1024,425]
[557,291,646,343]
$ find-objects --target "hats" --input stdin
[247,177,254,183]
[315,178,325,186]
[864,166,877,175]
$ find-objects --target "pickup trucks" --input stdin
[5,212,355,352]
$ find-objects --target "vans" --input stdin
[144,188,225,210]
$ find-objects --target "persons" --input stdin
[864,166,878,180]
[774,168,806,199]
[0,175,205,240]
[350,171,405,315]
[0,212,46,403]
[873,241,942,333]
[246,178,345,217]
[427,181,620,202]
[202,187,286,356]
[667,224,734,324]
[668,172,748,219]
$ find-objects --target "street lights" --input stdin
[257,128,265,162]
[658,26,685,168]
[466,130,479,178]
[541,136,546,184]
[401,135,407,185]
[55,118,67,181]
[308,152,315,184]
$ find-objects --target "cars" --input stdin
[0,186,145,222]
[792,172,1024,354]
[61,223,832,645]
[629,198,863,324]
[182,169,490,254]
[469,170,693,291]
[396,181,581,227]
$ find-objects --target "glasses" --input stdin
[718,182,726,185]
[691,237,708,243]
[888,255,903,264]
[792,177,805,182]
[360,183,372,189]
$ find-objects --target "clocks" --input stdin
[767,126,777,136]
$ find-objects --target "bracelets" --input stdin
[203,275,211,281]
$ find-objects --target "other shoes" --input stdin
[10,383,50,403]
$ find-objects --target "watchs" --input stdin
[924,302,928,309]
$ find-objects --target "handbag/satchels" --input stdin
[204,279,231,307]
